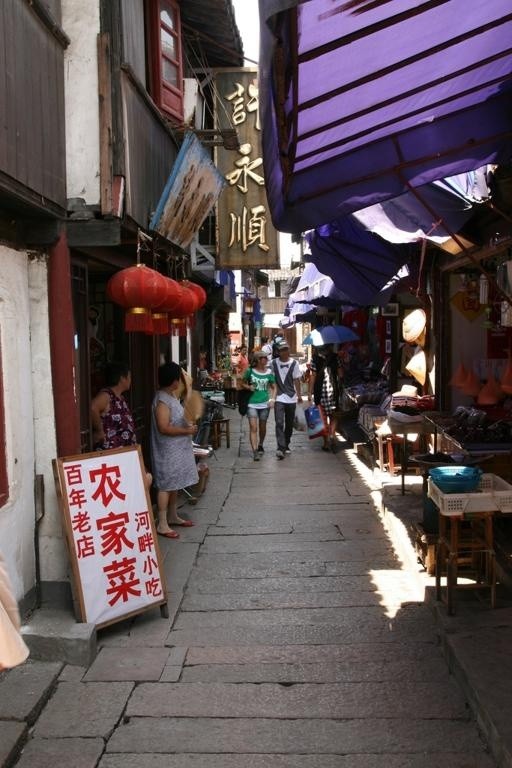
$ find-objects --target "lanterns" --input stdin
[145,275,182,337]
[177,279,208,332]
[106,263,169,333]
[170,285,198,338]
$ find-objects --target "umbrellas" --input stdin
[301,324,362,353]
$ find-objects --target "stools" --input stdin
[434,509,496,614]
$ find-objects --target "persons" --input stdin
[199,334,288,391]
[90,362,154,492]
[308,343,344,451]
[149,361,201,539]
[240,350,277,462]
[269,342,304,459]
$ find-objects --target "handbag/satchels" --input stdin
[237,389,252,415]
[304,403,328,439]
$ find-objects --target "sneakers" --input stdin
[276,447,290,458]
[254,445,264,460]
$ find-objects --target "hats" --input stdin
[253,351,270,358]
[275,340,289,350]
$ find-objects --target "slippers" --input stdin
[168,520,192,526]
[157,529,179,538]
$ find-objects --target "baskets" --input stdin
[426,467,512,516]
